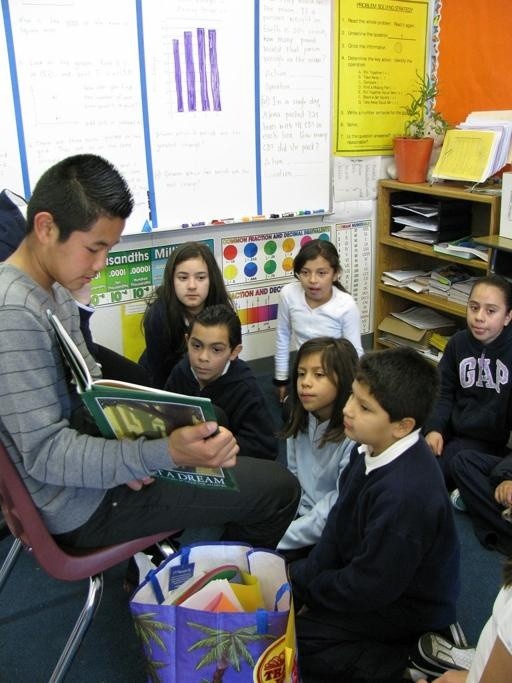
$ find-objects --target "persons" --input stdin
[0,189,184,594]
[274,337,361,564]
[161,303,280,462]
[421,273,512,512]
[415,556,512,683]
[284,345,477,683]
[138,241,232,390]
[449,449,512,556]
[273,238,363,437]
[1,153,303,552]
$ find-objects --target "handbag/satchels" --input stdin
[128,540,300,682]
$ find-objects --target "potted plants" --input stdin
[394,69,450,184]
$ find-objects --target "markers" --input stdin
[182,209,324,229]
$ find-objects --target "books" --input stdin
[45,309,241,494]
[377,197,512,365]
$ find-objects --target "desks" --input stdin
[473,234,512,287]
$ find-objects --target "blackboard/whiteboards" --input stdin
[0,0,335,243]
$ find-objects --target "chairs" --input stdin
[0,439,186,683]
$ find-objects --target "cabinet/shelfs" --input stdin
[374,178,502,367]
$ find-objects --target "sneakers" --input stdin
[450,487,467,511]
[408,621,477,676]
